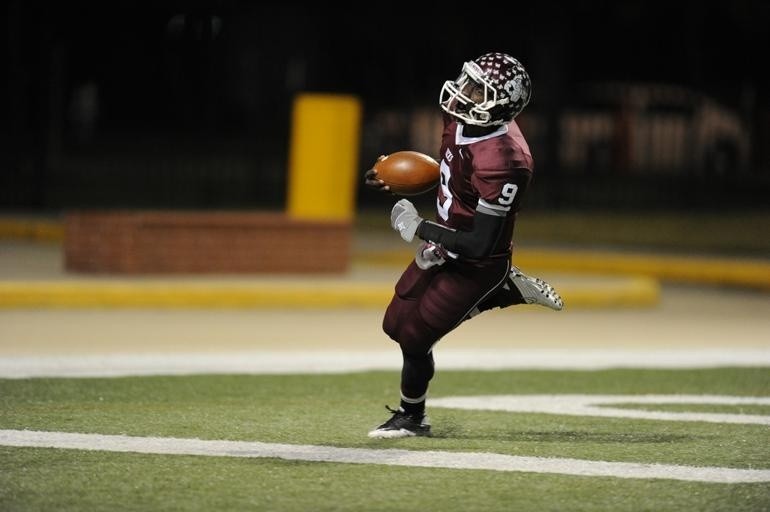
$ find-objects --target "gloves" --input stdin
[390,199,424,242]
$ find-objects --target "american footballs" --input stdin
[372,150,441,196]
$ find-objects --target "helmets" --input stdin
[439,52,533,128]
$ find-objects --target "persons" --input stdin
[362,51,566,442]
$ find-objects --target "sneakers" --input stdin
[508,264,563,310]
[368,405,431,438]
